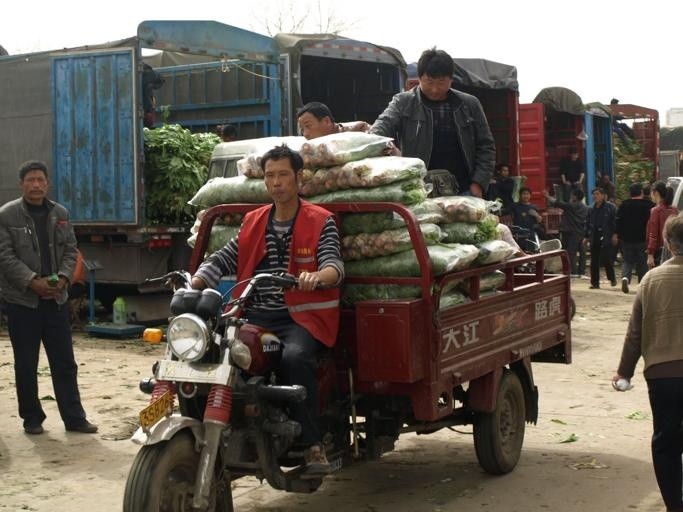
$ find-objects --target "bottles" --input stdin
[48,274,68,305]
[112,296,127,324]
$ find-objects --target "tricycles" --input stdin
[123,200,575,512]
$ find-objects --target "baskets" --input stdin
[539,239,563,272]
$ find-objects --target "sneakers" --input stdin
[66,419,97,432]
[303,442,330,475]
[25,424,43,434]
[621,277,629,293]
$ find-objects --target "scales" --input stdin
[81,256,147,337]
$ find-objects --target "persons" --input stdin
[0,160,99,434]
[216,123,237,142]
[367,46,496,199]
[487,147,683,293]
[176,143,343,475]
[611,211,682,510]
[610,98,639,148]
[141,62,164,129]
[297,101,400,156]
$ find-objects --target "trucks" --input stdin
[582,101,614,215]
[0,18,289,314]
[140,33,407,151]
[401,56,520,232]
[606,101,660,206]
[520,85,586,239]
[658,149,682,185]
